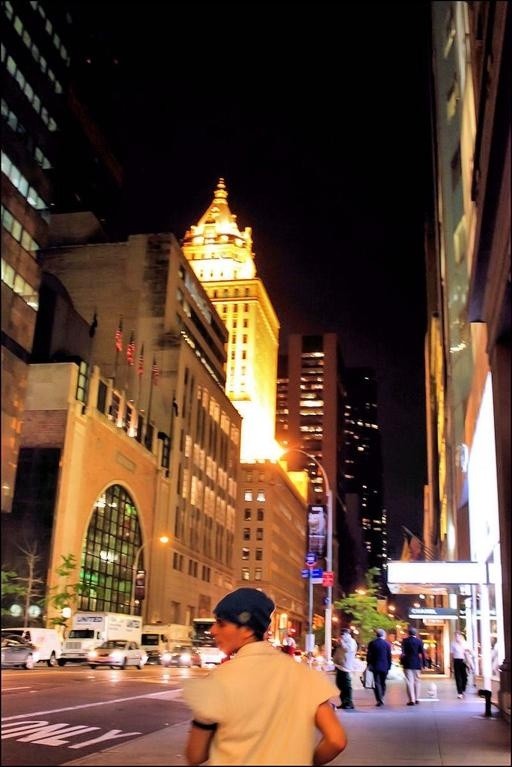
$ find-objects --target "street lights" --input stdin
[263,442,333,667]
[130,536,168,616]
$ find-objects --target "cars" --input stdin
[1,632,40,669]
[161,646,202,668]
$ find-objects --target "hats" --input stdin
[213,589,274,635]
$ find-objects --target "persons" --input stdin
[283,625,298,649]
[399,626,428,706]
[450,632,474,700]
[365,629,393,708]
[331,628,358,709]
[180,587,348,766]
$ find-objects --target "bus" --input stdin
[192,618,272,668]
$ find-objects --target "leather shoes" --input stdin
[343,705,353,709]
[407,702,414,705]
[376,701,384,707]
[337,705,343,709]
[416,701,419,704]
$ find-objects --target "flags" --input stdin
[400,534,422,561]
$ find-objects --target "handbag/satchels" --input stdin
[359,669,375,689]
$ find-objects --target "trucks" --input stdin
[59,612,193,674]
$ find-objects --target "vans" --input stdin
[2,628,62,666]
